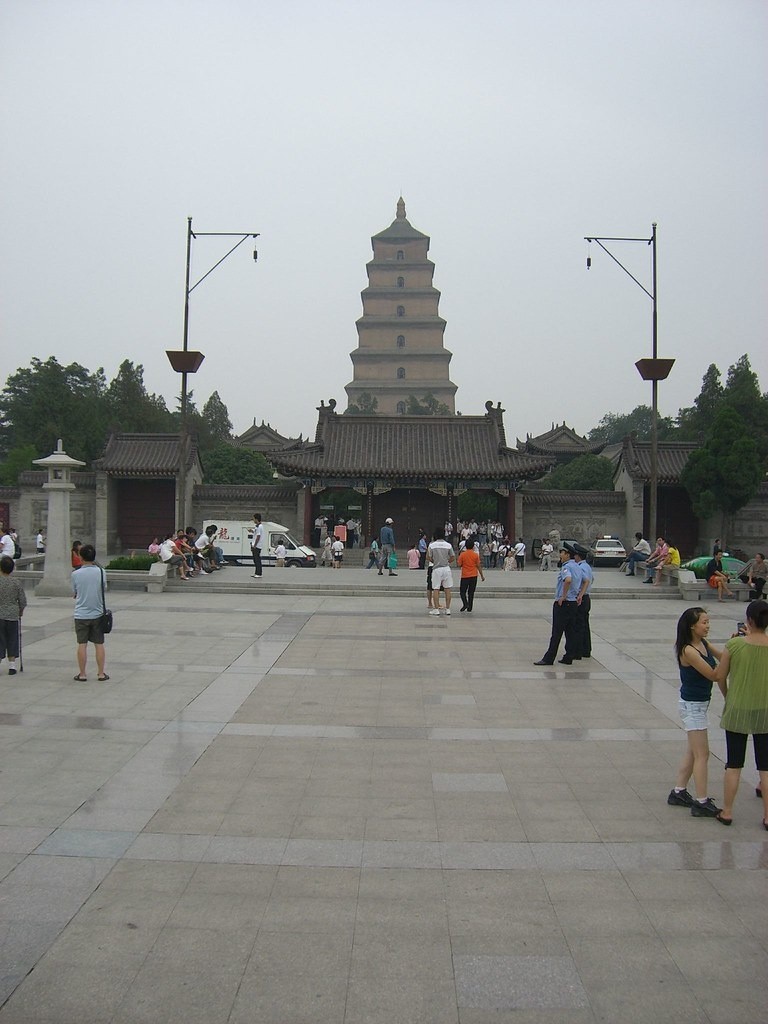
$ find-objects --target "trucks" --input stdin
[202,520,316,568]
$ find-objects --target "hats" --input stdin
[562,541,578,557]
[385,518,394,524]
[573,542,590,555]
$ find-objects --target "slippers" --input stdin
[761,818,768,830]
[73,673,88,681]
[715,810,732,825]
[97,673,110,681]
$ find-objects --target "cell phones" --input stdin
[738,622,746,638]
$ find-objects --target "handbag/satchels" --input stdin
[387,552,397,568]
[13,544,22,559]
[368,551,375,560]
[102,608,113,634]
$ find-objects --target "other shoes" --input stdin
[623,557,663,586]
[251,574,263,578]
[558,658,573,665]
[8,669,18,675]
[727,592,736,597]
[377,572,384,575]
[389,572,399,576]
[180,561,231,580]
[755,788,762,798]
[718,599,726,603]
[533,659,554,665]
[427,604,472,615]
[574,653,591,660]
[750,582,756,589]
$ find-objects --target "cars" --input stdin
[532,538,578,559]
[681,552,749,579]
[589,536,627,568]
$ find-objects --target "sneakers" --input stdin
[691,797,723,818]
[667,788,695,808]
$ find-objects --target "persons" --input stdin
[668,607,739,818]
[564,543,595,660]
[534,541,590,665]
[740,553,768,602]
[248,513,264,578]
[458,539,485,612]
[36,528,47,553]
[539,539,554,571]
[622,532,651,576]
[716,600,768,829]
[0,527,18,560]
[706,549,735,602]
[365,535,389,569]
[714,539,720,552]
[643,535,671,583]
[73,541,97,571]
[273,540,287,567]
[0,554,26,675]
[444,518,518,572]
[314,514,363,568]
[513,538,526,571]
[429,527,455,615]
[407,527,427,569]
[148,525,229,580]
[71,545,110,681]
[425,533,444,609]
[378,517,398,576]
[651,538,680,585]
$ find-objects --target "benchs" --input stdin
[151,562,180,578]
[632,560,695,585]
[681,581,768,603]
[10,553,45,571]
